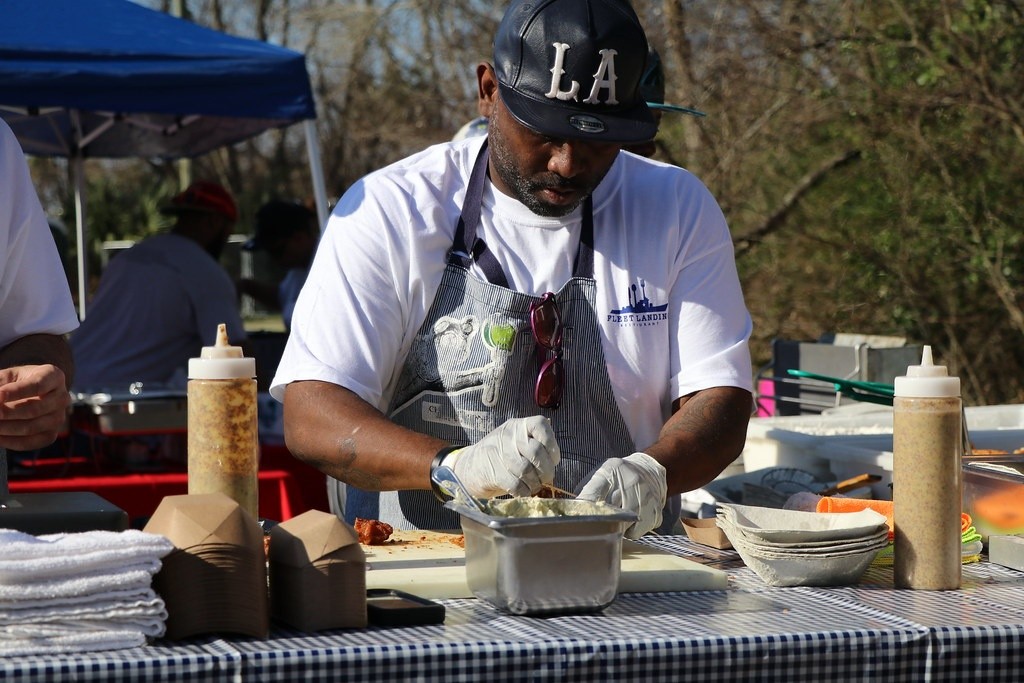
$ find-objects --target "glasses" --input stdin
[529,290,566,412]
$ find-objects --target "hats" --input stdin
[492,0,667,144]
[162,180,238,223]
[239,198,310,254]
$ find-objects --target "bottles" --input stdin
[186,323,258,523]
[893,345,963,591]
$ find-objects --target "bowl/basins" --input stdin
[714,501,891,587]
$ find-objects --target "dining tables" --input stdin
[1,535,1024,683]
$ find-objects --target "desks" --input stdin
[7,444,329,529]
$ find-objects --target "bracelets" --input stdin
[429,445,468,504]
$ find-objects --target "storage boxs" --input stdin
[679,502,891,587]
[142,493,367,641]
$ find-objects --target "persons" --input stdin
[239,200,320,331]
[67,179,247,405]
[268,1,759,535]
[0,115,80,450]
